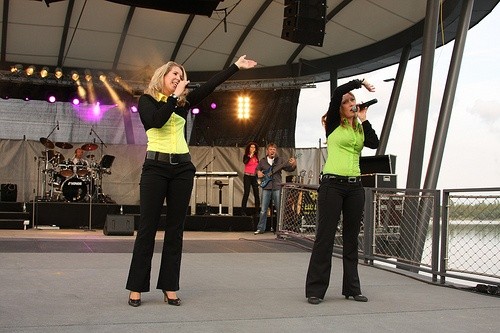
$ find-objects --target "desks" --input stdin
[193,171,238,214]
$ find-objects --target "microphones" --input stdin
[352,99,378,112]
[186,84,201,88]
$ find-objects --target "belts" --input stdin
[145,151,192,165]
[321,174,361,184]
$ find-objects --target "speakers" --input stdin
[104,214,135,235]
[281,0,327,48]
[109,0,224,17]
[1,184,18,202]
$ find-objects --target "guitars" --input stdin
[256,153,303,188]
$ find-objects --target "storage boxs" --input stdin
[361,173,402,229]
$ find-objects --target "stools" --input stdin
[211,183,231,217]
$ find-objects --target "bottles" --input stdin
[120,205,123,214]
[23,202,26,212]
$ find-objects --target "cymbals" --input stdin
[44,150,65,166]
[81,143,99,151]
[86,154,95,158]
[55,142,73,149]
[40,137,55,149]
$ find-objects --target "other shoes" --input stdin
[255,212,260,217]
[254,230,265,235]
[241,211,247,217]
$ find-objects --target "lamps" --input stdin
[10,65,121,84]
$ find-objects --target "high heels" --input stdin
[162,289,182,306]
[128,292,142,307]
[307,297,320,304]
[345,293,368,302]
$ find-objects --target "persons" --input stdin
[305,78,381,305]
[240,142,261,216]
[71,148,96,197]
[253,143,297,234]
[125,52,258,306]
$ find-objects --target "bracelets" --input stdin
[169,94,179,101]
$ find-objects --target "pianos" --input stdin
[190,171,239,216]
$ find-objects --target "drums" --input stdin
[60,159,75,178]
[48,172,66,189]
[61,174,88,203]
[76,160,90,178]
